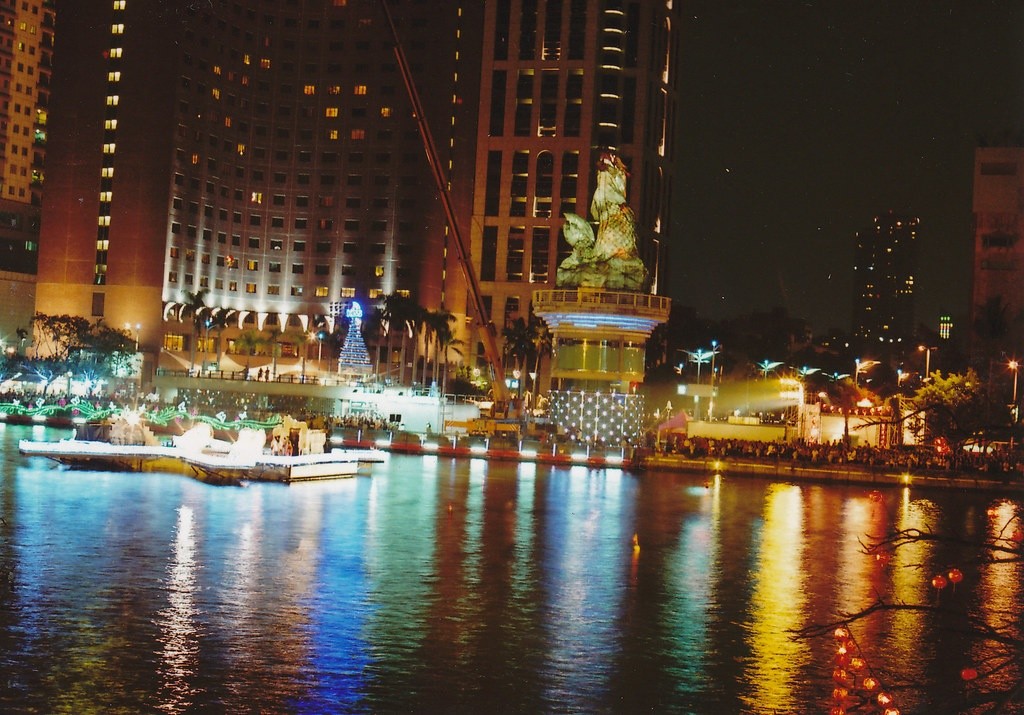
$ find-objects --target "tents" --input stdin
[657,409,691,443]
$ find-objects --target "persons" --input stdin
[265,366,269,381]
[240,365,248,380]
[257,367,263,381]
[336,410,403,431]
[426,422,431,434]
[683,431,1017,473]
